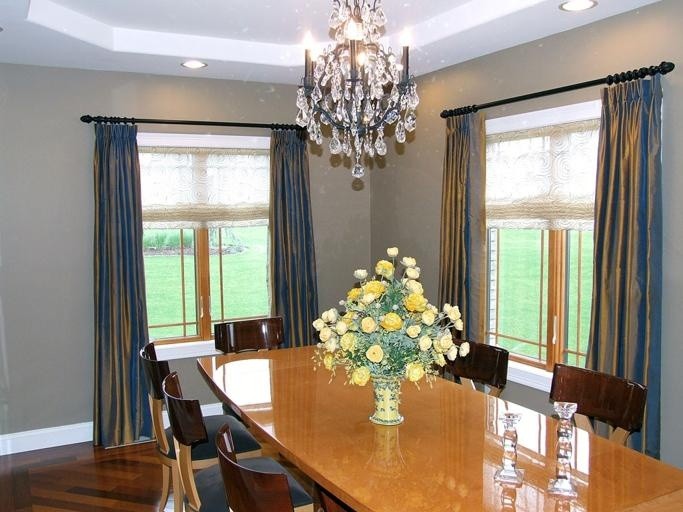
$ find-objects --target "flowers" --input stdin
[309,243,472,392]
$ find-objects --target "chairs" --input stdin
[546,360,648,447]
[215,424,296,510]
[141,339,263,512]
[444,337,509,397]
[212,316,288,355]
[161,368,313,512]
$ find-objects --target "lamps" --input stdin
[295,0,421,181]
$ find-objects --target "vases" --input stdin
[368,363,407,429]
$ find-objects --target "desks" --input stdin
[195,345,683,512]
